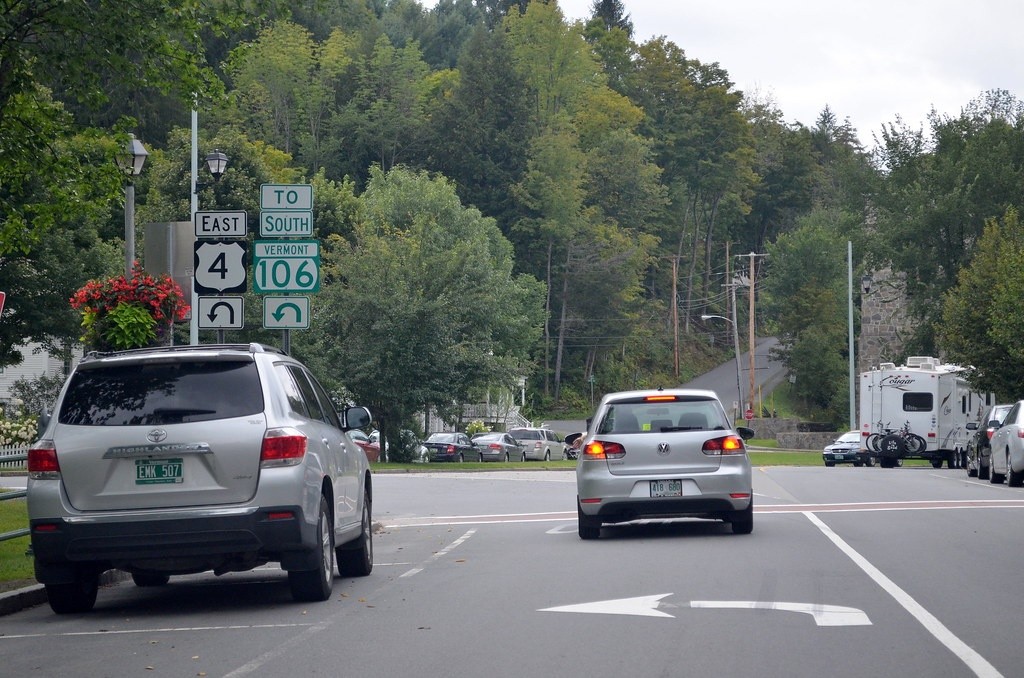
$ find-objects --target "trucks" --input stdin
[859,356,996,469]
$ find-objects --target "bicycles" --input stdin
[866,420,927,454]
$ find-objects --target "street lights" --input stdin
[701,283,743,420]
[190,91,229,346]
[848,240,873,431]
[114,133,150,285]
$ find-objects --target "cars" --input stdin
[565,387,755,538]
[369,429,430,463]
[424,432,483,462]
[507,427,568,462]
[470,432,526,462]
[988,400,1024,487]
[822,430,903,467]
[966,405,1014,479]
[345,429,382,462]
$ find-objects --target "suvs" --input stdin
[26,342,373,614]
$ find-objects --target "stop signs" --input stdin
[745,409,754,420]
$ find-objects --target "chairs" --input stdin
[615,414,640,430]
[677,412,708,428]
